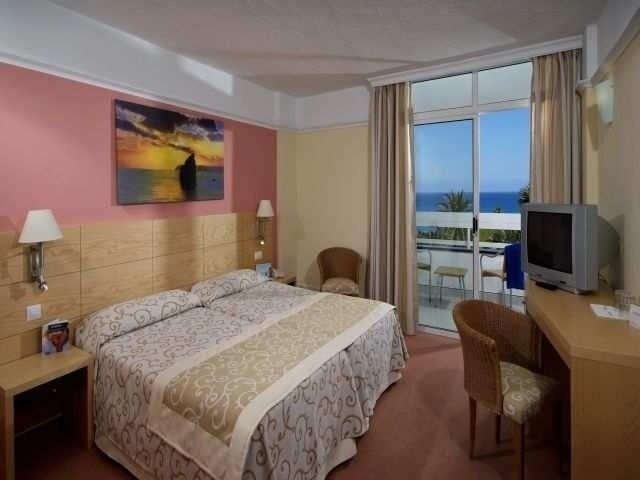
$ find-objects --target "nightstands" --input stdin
[0,344,93,480]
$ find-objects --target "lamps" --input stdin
[256,199,274,247]
[19,209,63,292]
[595,79,613,125]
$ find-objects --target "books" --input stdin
[42,318,70,355]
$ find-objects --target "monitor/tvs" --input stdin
[520,202,621,296]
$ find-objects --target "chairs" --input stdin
[418,249,434,304]
[317,246,363,297]
[433,266,467,307]
[451,299,565,480]
[480,251,512,309]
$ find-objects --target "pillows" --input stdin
[82,289,201,345]
[190,268,269,303]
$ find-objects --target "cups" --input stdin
[615,290,625,308]
[624,288,631,310]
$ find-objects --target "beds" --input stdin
[74,269,402,475]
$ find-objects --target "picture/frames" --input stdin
[112,98,226,206]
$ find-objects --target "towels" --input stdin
[504,243,524,289]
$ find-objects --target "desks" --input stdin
[526,278,639,479]
